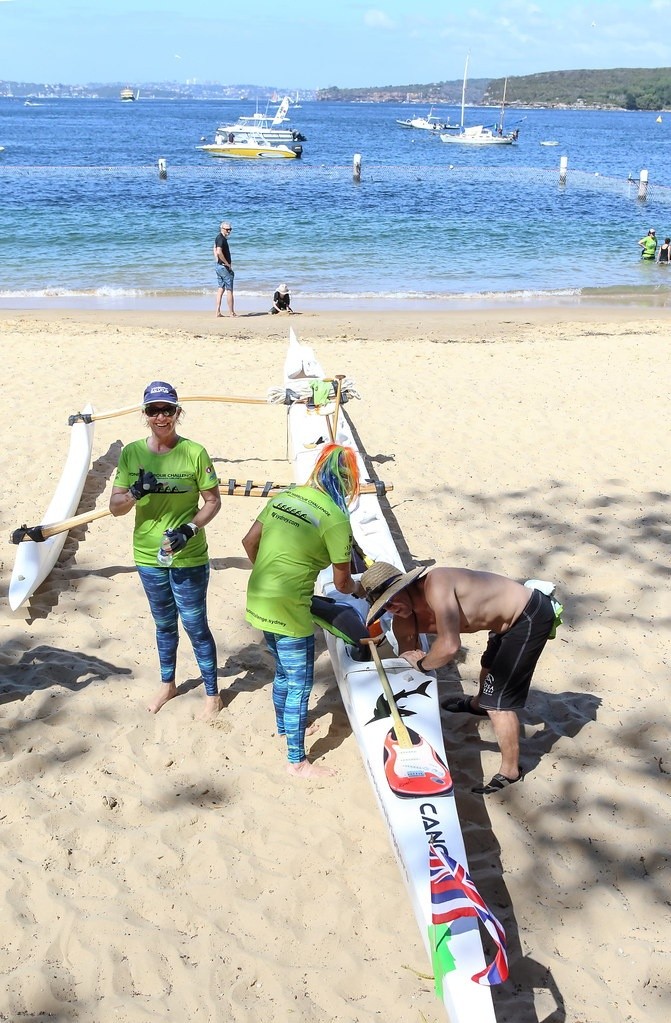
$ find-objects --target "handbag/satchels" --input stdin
[441,697,483,715]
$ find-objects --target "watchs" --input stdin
[187,522,199,537]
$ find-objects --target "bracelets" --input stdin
[417,656,431,673]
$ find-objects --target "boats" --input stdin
[281,325,496,1023]
[217,94,307,143]
[195,139,303,159]
[120,87,136,102]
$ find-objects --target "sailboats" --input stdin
[394,49,470,131]
[439,75,528,144]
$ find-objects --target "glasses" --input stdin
[145,405,177,417]
[223,228,232,231]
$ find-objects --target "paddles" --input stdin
[358,637,454,799]
[328,374,346,444]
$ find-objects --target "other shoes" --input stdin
[472,766,524,794]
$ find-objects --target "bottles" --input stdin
[157,527,174,567]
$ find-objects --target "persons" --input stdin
[656,237,671,265]
[213,222,239,318]
[242,445,366,780]
[359,562,555,794]
[268,284,291,315]
[109,382,224,720]
[638,229,658,260]
[228,133,235,143]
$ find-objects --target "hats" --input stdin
[276,284,290,295]
[144,381,177,404]
[360,562,426,627]
[649,229,655,233]
